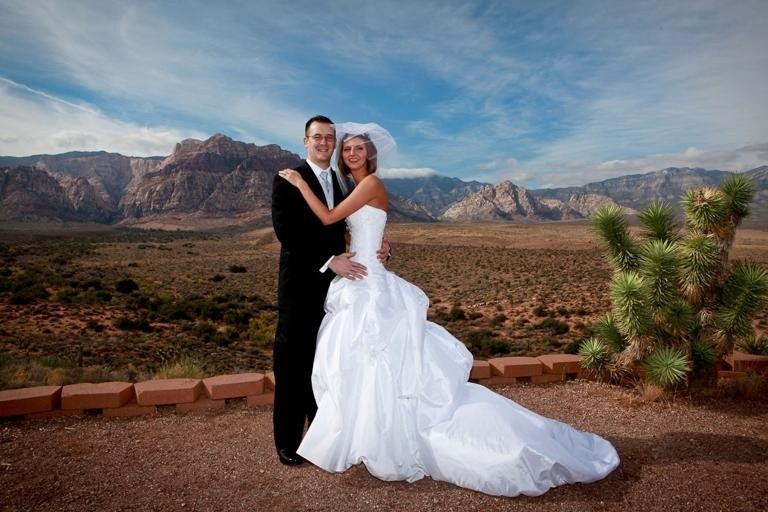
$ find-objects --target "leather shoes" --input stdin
[277,447,304,465]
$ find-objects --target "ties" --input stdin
[320,172,330,193]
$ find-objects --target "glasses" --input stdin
[306,134,334,141]
